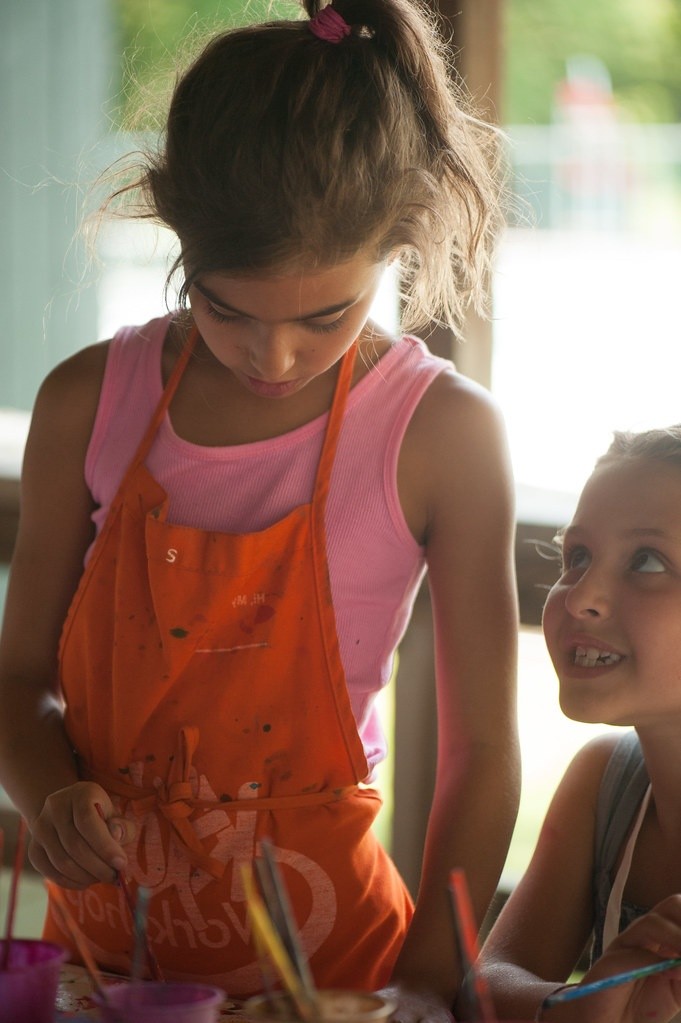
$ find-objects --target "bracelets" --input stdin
[532,983,579,1023]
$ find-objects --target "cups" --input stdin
[93,981,226,1022]
[246,989,399,1022]
[0,938,71,1022]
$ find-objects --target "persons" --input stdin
[0,0,523,1022]
[452,425,681,1023]
